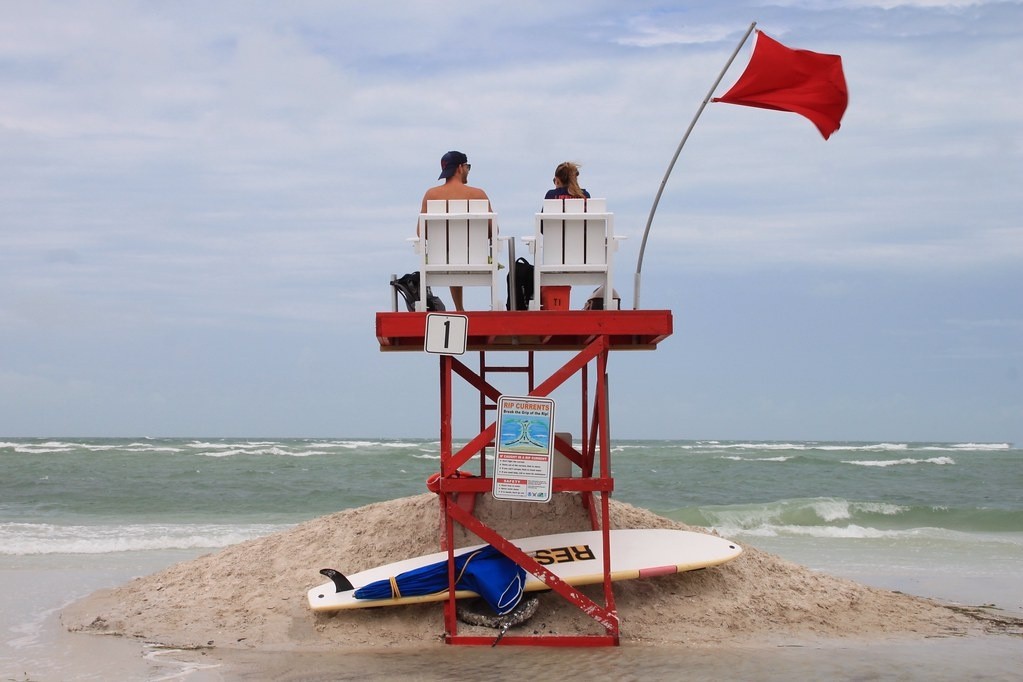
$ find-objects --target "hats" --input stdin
[438,150,468,181]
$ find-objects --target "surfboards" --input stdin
[306,528,745,612]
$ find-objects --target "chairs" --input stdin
[519,197,628,310]
[405,199,512,312]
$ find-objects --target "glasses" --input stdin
[462,164,471,171]
[553,178,555,184]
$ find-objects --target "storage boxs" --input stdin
[541,286,572,311]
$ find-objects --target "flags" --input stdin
[713,29,848,140]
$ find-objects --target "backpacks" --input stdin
[389,271,446,312]
[505,257,542,311]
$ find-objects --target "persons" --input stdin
[541,162,591,264]
[417,150,499,311]
